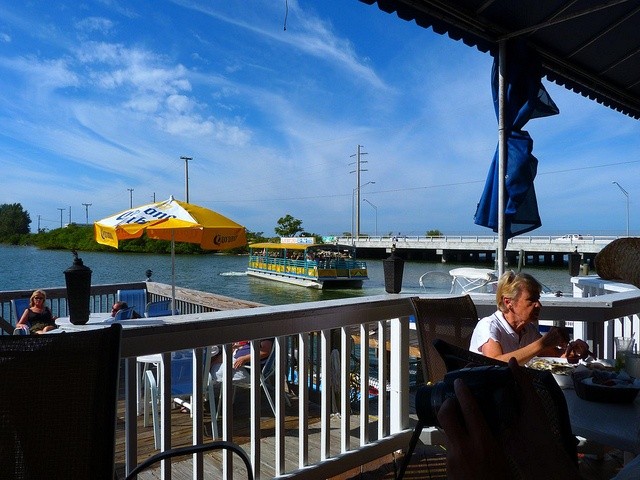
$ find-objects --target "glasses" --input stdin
[34,296,43,299]
[112,306,118,312]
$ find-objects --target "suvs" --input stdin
[555,234,584,241]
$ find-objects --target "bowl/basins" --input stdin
[551,365,576,390]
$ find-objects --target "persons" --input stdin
[471,270,589,366]
[253,247,353,267]
[102,301,128,326]
[204,338,273,438]
[17,290,57,336]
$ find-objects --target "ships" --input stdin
[246,242,370,290]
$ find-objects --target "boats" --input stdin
[385,266,574,339]
[287,348,392,405]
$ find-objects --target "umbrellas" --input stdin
[93,194,248,315]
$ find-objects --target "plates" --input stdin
[525,356,587,372]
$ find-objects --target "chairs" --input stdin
[1,322,123,480]
[216,342,275,426]
[443,361,580,480]
[117,288,147,317]
[11,299,35,334]
[143,350,219,450]
[397,297,479,478]
[144,300,172,318]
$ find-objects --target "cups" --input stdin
[613,337,635,368]
[623,350,640,379]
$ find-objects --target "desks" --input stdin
[55,312,117,326]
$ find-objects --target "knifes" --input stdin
[585,348,597,361]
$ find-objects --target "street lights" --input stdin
[363,198,377,236]
[612,181,630,235]
[351,181,376,242]
